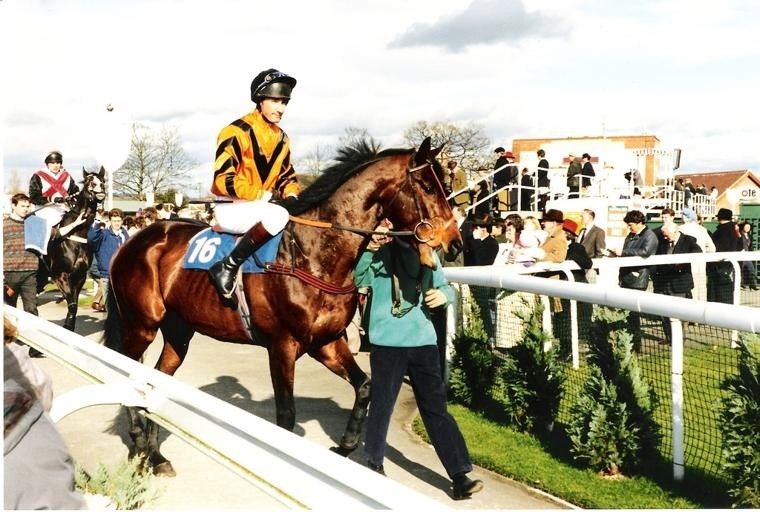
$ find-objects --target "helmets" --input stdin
[45,152,63,163]
[251,69,296,102]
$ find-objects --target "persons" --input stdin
[451,207,605,363]
[122,202,182,237]
[352,222,486,499]
[612,208,760,353]
[3,152,128,357]
[4,318,90,508]
[667,178,717,204]
[447,147,595,222]
[206,67,301,310]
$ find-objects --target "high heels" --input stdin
[749,285,760,291]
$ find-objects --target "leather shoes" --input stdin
[92,302,99,309]
[368,460,385,474]
[29,347,40,357]
[99,305,105,312]
[453,477,484,500]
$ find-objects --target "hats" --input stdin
[715,208,734,220]
[503,152,515,158]
[470,213,493,226]
[447,161,457,169]
[680,208,696,220]
[543,209,564,223]
[562,219,578,237]
[495,147,504,152]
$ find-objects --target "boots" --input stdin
[208,221,274,307]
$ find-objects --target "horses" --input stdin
[99,136,465,479]
[36,164,106,332]
